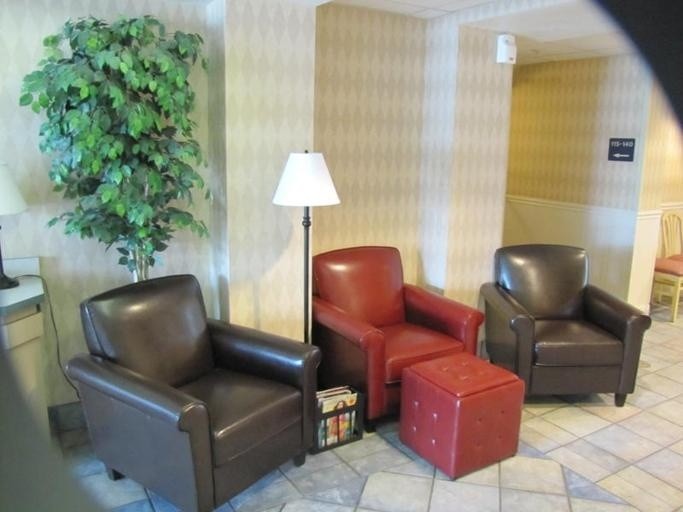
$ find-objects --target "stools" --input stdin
[652,258,683,322]
[396,353,527,482]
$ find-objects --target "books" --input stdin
[314,385,358,451]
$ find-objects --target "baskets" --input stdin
[314,387,362,452]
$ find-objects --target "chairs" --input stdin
[65,272,323,512]
[657,210,683,305]
[308,243,485,435]
[480,241,651,407]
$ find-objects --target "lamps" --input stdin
[0,160,29,289]
[274,148,341,343]
